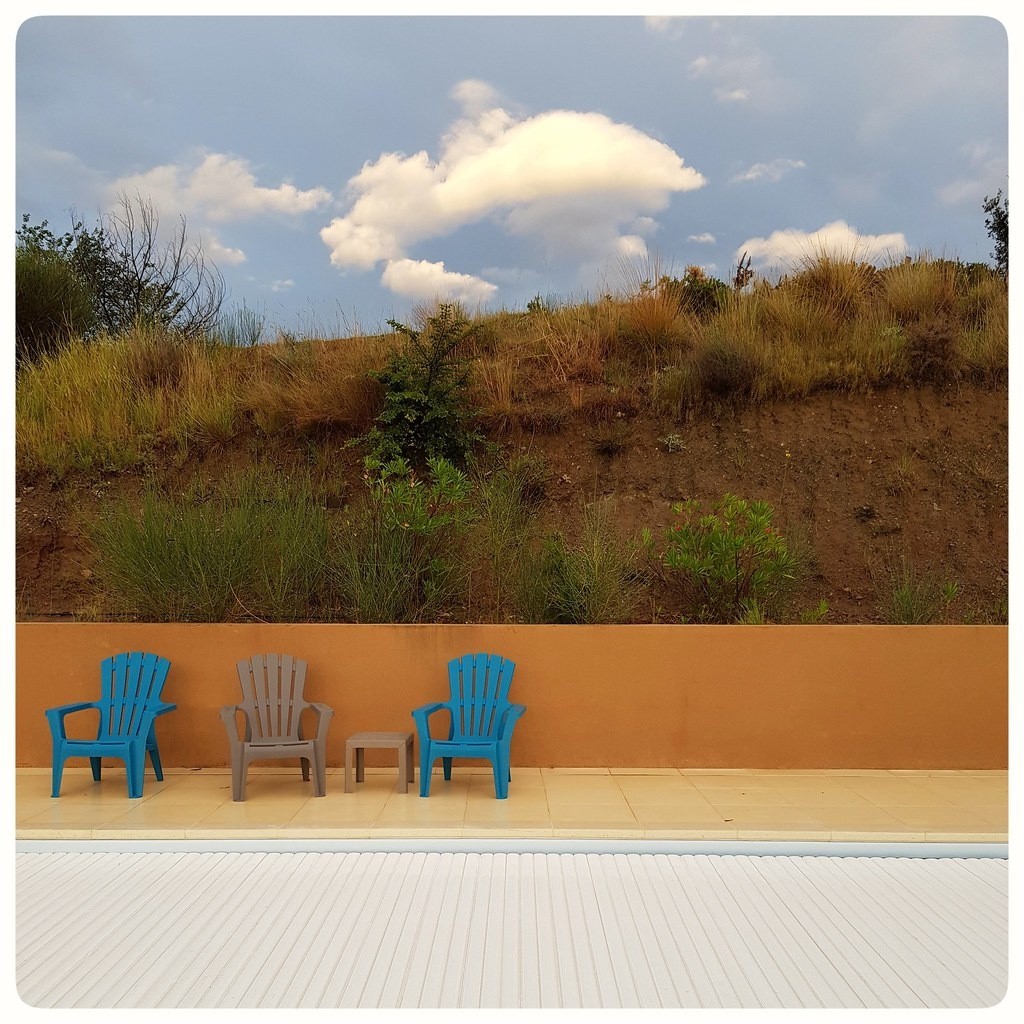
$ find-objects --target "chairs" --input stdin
[412,652,527,799]
[44,651,179,798]
[221,652,334,802]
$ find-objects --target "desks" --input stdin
[343,731,412,795]
